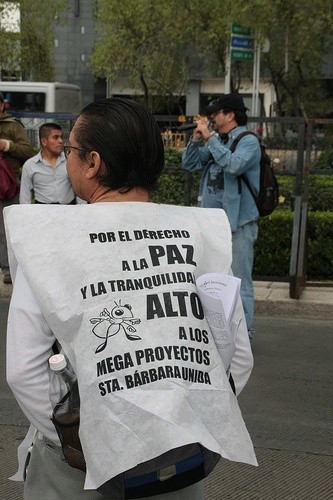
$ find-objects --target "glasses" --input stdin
[63,140,87,160]
[214,111,223,115]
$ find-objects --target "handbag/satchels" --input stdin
[0,156,19,201]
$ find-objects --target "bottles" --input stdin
[48,354,86,469]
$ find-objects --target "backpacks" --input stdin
[49,369,238,485]
[230,131,280,218]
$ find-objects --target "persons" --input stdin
[5,97,254,500]
[164,129,171,140]
[0,92,35,284]
[182,93,261,341]
[19,123,77,205]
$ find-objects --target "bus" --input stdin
[0,81,82,151]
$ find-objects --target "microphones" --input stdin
[176,120,210,132]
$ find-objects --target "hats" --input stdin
[201,92,250,116]
